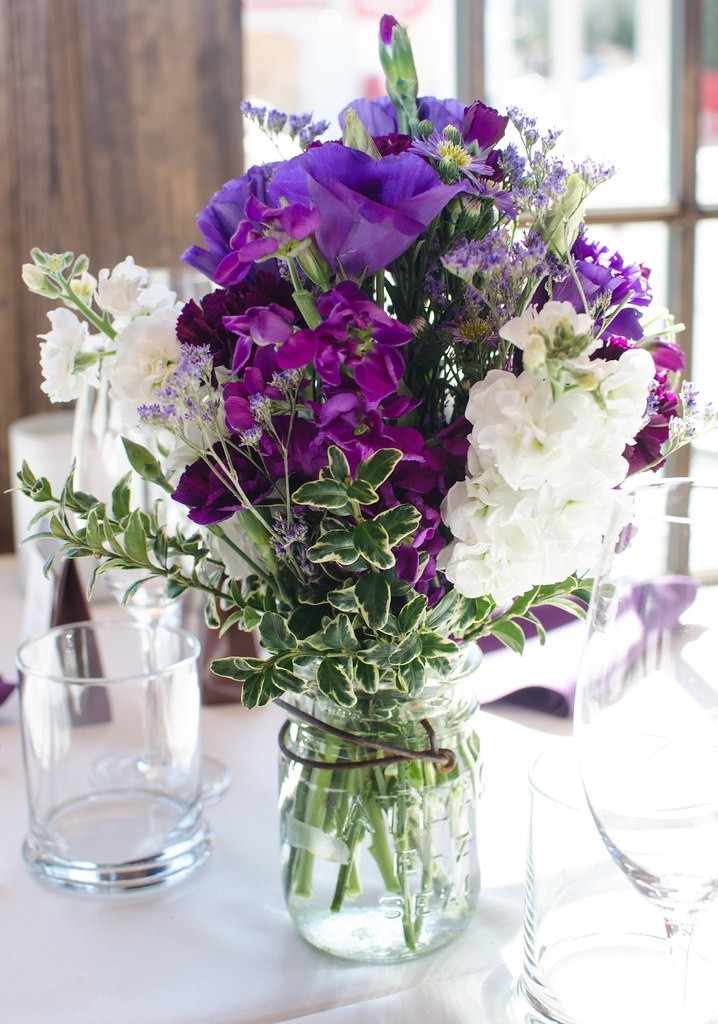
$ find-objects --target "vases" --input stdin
[271,639,487,969]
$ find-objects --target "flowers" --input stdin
[4,13,718,953]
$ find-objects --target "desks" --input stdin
[0,413,718,1023]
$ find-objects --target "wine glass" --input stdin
[575,477,718,1023]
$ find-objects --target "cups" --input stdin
[9,414,183,637]
[12,619,211,892]
[516,737,716,1022]
